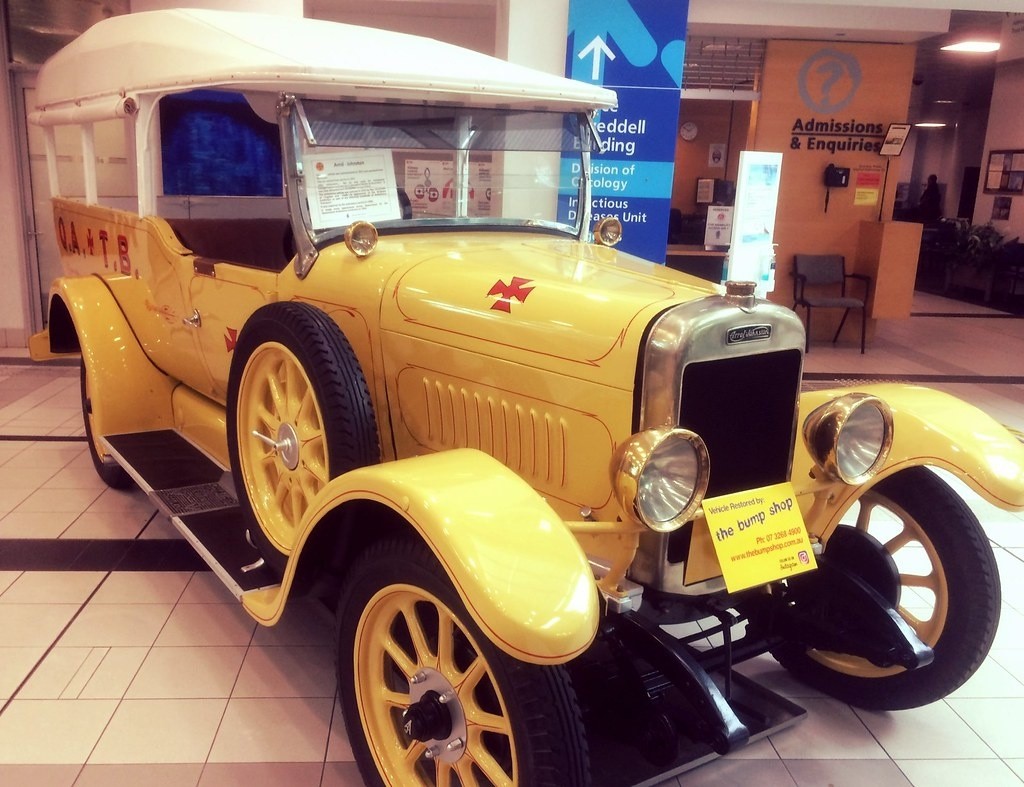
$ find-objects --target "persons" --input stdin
[920,174,938,208]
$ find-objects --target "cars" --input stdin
[27,7,1024,787]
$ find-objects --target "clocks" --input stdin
[680,123,698,141]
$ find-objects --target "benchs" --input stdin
[162,214,295,278]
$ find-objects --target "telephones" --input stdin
[823,163,851,187]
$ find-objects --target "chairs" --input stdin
[787,254,874,354]
